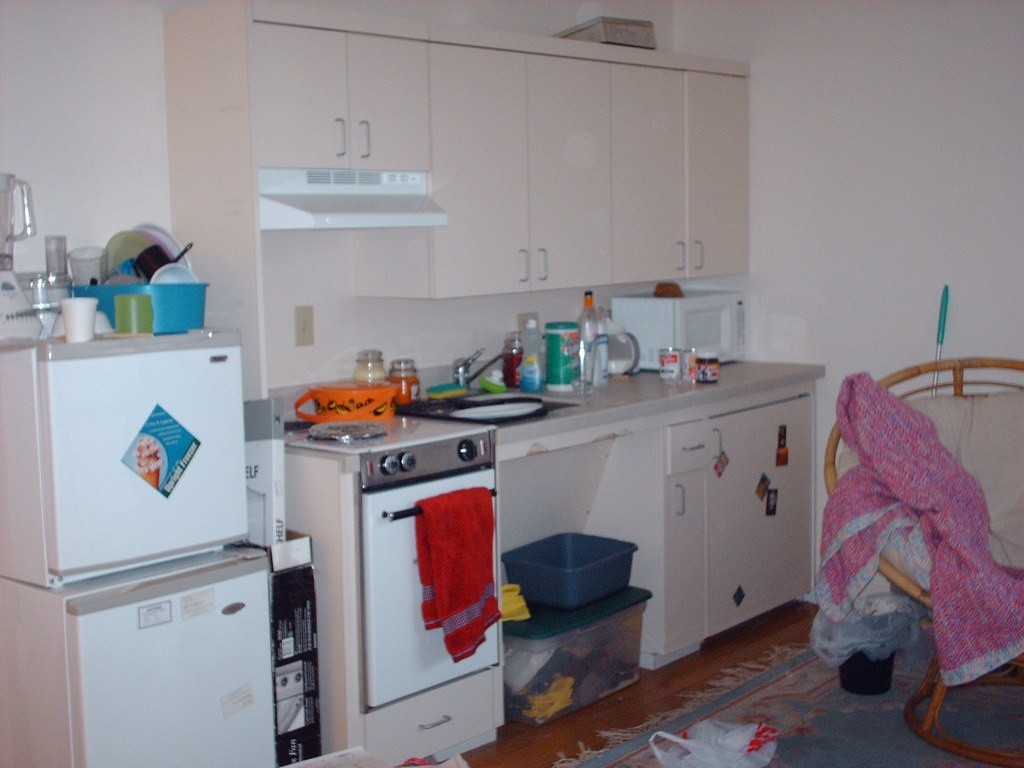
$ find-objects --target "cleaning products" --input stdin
[521,355,539,392]
[544,321,582,392]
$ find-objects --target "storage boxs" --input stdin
[228,533,321,768]
[504,586,652,726]
[552,15,656,49]
[502,534,636,609]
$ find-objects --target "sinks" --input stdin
[467,399,579,416]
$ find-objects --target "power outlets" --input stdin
[296,305,314,345]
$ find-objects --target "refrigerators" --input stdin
[0,328,278,768]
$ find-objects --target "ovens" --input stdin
[285,464,505,762]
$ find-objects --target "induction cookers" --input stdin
[282,416,497,490]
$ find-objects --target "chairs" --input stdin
[824,357,1023,768]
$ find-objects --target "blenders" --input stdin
[1,173,46,345]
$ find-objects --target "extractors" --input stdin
[256,167,449,233]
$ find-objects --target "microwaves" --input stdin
[611,287,743,371]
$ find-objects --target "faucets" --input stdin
[454,347,519,387]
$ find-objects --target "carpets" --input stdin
[555,632,1024,768]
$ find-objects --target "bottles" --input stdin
[506,290,719,396]
[352,349,386,382]
[388,358,419,406]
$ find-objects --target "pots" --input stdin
[294,381,396,432]
[397,396,549,425]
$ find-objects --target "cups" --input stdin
[564,339,594,395]
[16,236,153,343]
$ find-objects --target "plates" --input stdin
[451,402,544,418]
[99,224,196,285]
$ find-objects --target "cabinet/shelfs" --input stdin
[242,396,285,551]
[503,396,811,672]
[285,454,501,764]
[163,0,749,299]
